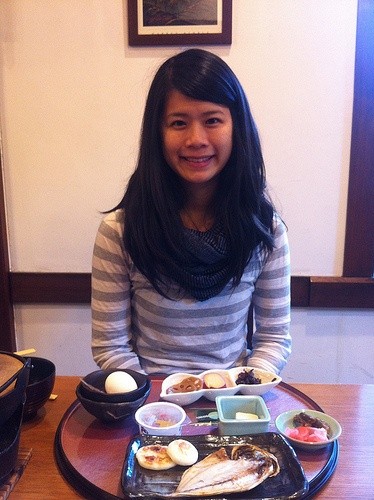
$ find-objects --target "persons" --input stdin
[91,48,291,378]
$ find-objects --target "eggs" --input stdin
[105,370,138,394]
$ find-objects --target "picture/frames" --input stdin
[127,0,232,48]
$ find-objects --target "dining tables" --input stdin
[0,375,374,500]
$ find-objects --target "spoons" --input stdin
[195,412,219,423]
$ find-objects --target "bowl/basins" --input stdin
[16,354,57,423]
[211,394,272,435]
[275,410,343,453]
[76,369,153,425]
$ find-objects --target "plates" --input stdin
[159,364,284,408]
[121,434,311,500]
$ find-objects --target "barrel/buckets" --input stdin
[0,352,31,483]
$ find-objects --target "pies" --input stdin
[167,439,198,466]
[135,445,177,470]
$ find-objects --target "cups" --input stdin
[135,399,188,438]
